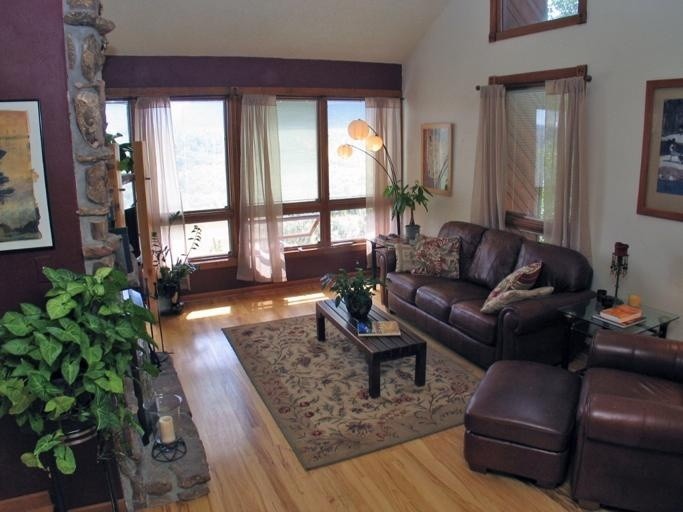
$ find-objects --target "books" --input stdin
[593,313,646,328]
[600,305,642,324]
[358,320,401,337]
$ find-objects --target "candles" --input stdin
[157,414,175,443]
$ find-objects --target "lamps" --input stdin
[603,241,629,309]
[335,117,401,241]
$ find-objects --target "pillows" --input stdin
[480,286,555,315]
[479,258,543,308]
[392,243,413,272]
[406,236,462,282]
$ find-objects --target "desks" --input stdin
[556,294,679,365]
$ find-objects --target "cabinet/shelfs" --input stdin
[367,236,407,292]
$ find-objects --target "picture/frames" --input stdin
[0,97,56,254]
[417,122,453,198]
[631,76,683,223]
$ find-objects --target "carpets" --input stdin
[221,312,483,472]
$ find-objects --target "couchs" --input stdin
[378,220,594,374]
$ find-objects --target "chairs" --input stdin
[570,331,682,512]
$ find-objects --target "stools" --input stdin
[458,358,582,486]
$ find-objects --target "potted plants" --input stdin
[0,262,162,512]
[383,178,433,240]
[154,210,205,311]
[317,260,393,318]
